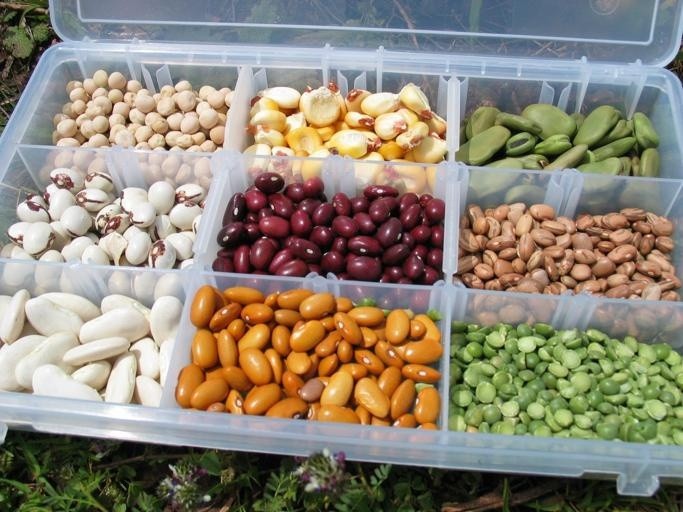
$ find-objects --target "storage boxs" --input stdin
[0,1,682,497]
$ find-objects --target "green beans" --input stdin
[0,69,683,444]
